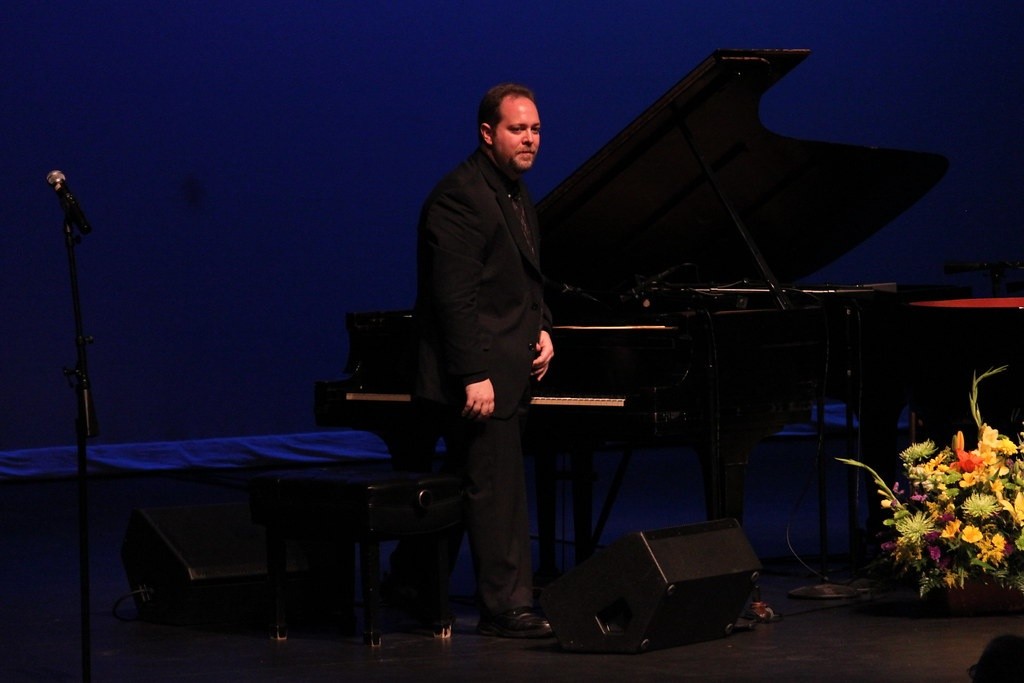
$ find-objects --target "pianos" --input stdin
[340,47,974,584]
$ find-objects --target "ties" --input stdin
[511,199,535,257]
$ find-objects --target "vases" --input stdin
[945,573,1024,614]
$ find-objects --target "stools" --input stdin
[247,464,487,650]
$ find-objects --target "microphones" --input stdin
[46,170,92,235]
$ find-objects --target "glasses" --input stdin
[967,664,980,680]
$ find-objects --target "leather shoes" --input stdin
[384,584,455,632]
[474,607,551,638]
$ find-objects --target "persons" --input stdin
[373,84,560,643]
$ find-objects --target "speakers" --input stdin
[539,517,762,655]
[120,501,316,626]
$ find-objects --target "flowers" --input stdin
[832,365,1024,597]
